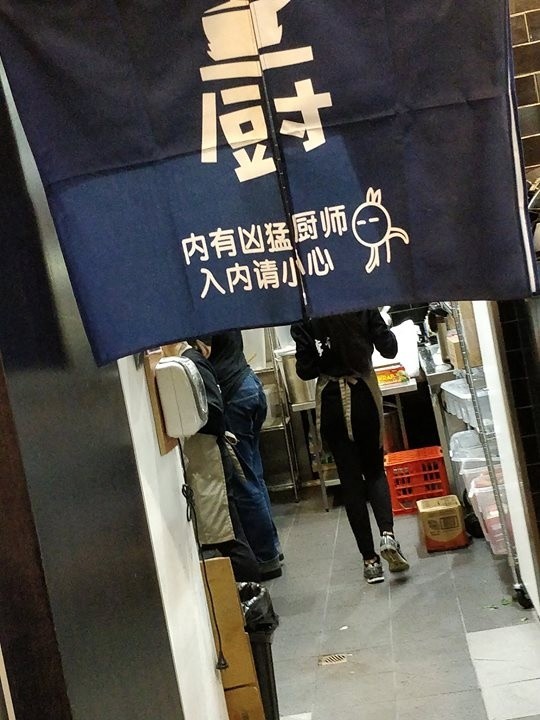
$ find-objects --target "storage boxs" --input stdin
[384,298,514,556]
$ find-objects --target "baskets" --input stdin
[383,444,449,516]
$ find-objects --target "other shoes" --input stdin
[259,566,283,581]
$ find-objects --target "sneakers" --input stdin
[378,535,409,572]
[363,556,385,584]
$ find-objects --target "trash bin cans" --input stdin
[235,581,278,720]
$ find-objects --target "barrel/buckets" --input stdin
[282,353,316,404]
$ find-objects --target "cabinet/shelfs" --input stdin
[291,377,418,510]
[251,325,299,503]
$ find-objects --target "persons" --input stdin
[287,305,410,583]
[163,330,285,585]
